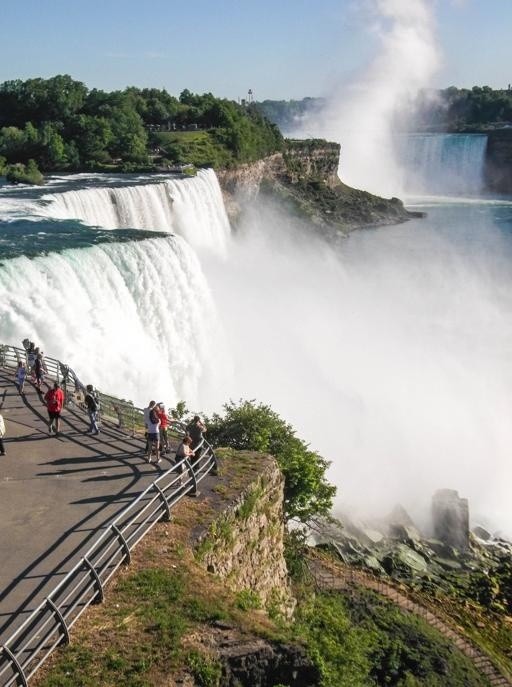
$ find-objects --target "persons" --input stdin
[143,400,162,463]
[83,384,102,436]
[154,402,171,454]
[45,383,65,437]
[0,414,9,457]
[174,437,195,489]
[144,431,149,452]
[184,415,208,473]
[13,339,48,396]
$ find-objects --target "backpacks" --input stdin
[88,392,100,411]
